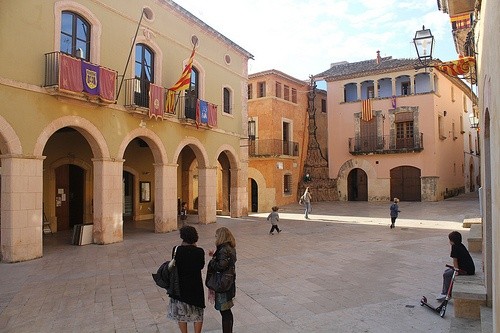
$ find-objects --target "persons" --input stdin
[390,198,402,229]
[302,187,312,219]
[180,202,189,227]
[437,231,475,301]
[206,227,237,333]
[167,226,205,333]
[266,206,282,236]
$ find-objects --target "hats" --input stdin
[272,206,279,210]
[394,201,398,203]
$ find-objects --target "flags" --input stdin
[164,50,195,114]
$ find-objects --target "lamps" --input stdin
[468,113,480,128]
[413,25,477,84]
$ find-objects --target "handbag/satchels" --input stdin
[167,245,179,272]
[205,251,231,294]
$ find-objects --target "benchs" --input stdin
[467,224,483,251]
[452,273,486,320]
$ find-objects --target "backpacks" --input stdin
[298,195,304,205]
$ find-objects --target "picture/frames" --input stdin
[140,181,151,202]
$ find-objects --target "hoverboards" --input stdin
[420,264,468,319]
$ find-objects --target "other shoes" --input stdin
[436,294,451,301]
[393,226,395,228]
[390,225,393,229]
[278,230,282,233]
[306,218,310,220]
[269,232,274,235]
